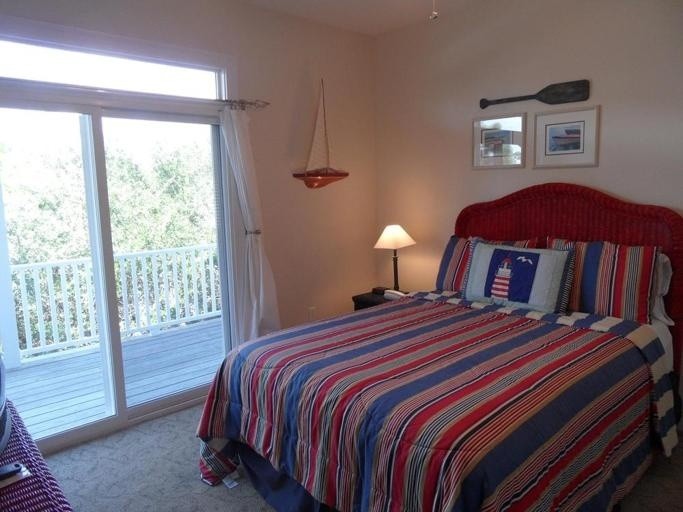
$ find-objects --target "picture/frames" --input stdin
[469,112,527,170]
[532,106,601,169]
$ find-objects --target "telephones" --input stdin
[383,289,404,300]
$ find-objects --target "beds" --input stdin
[195,183,683,512]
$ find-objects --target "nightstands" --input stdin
[352,292,411,311]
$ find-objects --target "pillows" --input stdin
[459,238,576,314]
[546,235,660,325]
[437,234,538,293]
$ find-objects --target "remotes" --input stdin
[0,462,21,480]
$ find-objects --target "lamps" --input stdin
[374,224,417,290]
